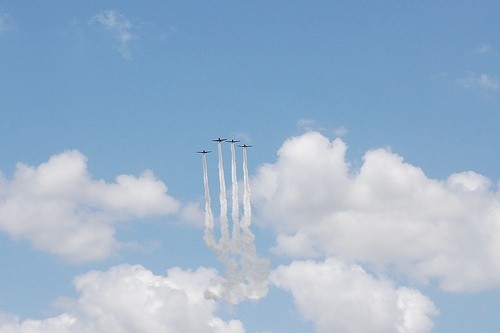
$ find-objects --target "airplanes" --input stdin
[196,149,212,154]
[238,144,251,148]
[225,140,240,143]
[212,138,227,142]
